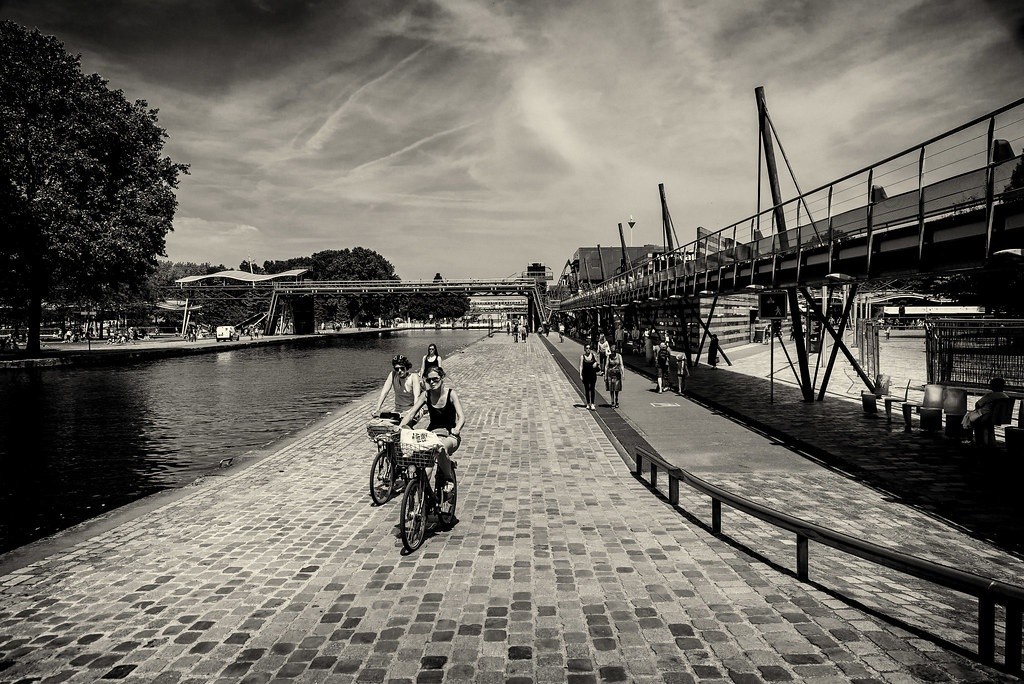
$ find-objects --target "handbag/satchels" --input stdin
[592,353,600,373]
[605,341,611,356]
[401,428,444,457]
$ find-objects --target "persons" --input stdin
[708,334,718,369]
[579,343,601,410]
[802,322,806,341]
[51,321,150,346]
[371,355,424,480]
[596,333,610,376]
[789,324,795,341]
[538,325,543,337]
[185,323,217,342]
[418,343,442,378]
[394,367,466,517]
[961,378,1009,443]
[506,322,529,343]
[558,323,565,343]
[885,327,891,340]
[605,345,625,409]
[615,324,690,396]
[236,326,264,340]
[545,322,550,337]
[570,321,596,349]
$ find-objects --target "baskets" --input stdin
[392,433,436,466]
[366,425,395,443]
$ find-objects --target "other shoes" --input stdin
[616,402,619,408]
[659,390,662,394]
[443,481,454,493]
[591,404,595,410]
[586,404,590,410]
[612,402,615,407]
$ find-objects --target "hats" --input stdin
[676,354,684,361]
[659,343,667,350]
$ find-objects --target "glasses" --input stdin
[425,377,442,383]
[394,366,405,371]
[429,348,436,351]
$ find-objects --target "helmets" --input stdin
[392,355,413,371]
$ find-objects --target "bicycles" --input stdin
[365,413,457,551]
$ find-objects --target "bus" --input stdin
[0,328,64,340]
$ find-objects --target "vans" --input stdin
[214,326,240,342]
